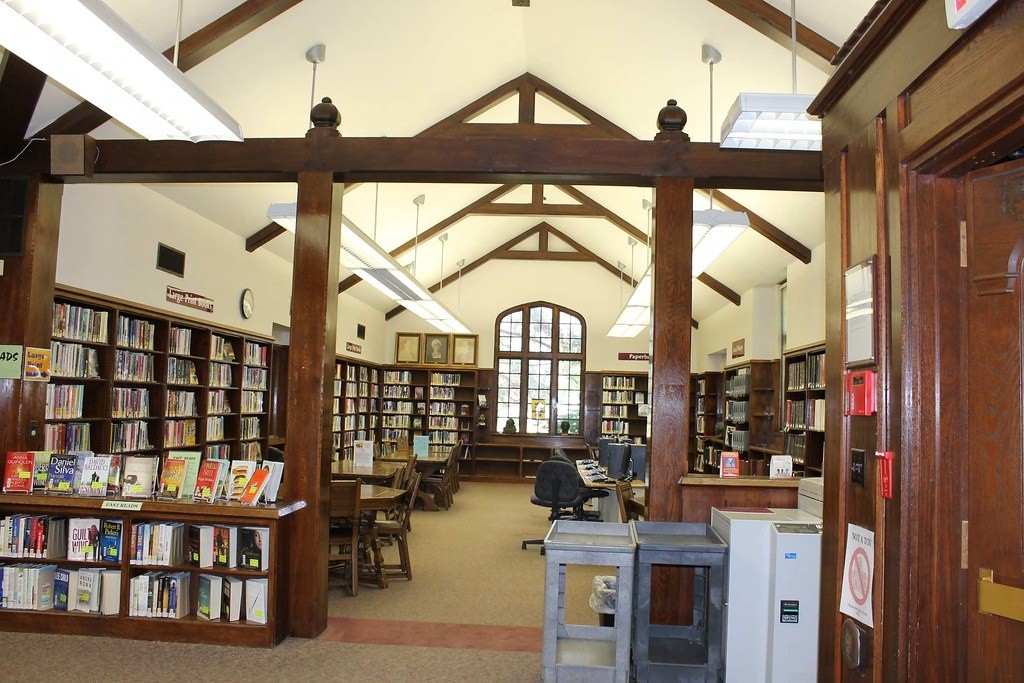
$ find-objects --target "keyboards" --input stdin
[582,459,608,482]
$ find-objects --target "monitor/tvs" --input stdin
[598,435,646,481]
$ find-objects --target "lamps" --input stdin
[0,0,243,141]
[268,183,472,334]
[606,45,750,338]
[719,0,822,151]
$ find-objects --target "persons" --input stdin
[457,341,472,362]
[84,525,100,562]
[244,530,262,564]
[427,339,445,361]
[399,339,416,361]
[216,530,225,556]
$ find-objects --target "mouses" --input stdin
[587,467,595,470]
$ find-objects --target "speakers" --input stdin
[49,133,95,179]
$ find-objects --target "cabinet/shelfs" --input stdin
[43,282,276,483]
[474,443,600,484]
[331,353,497,481]
[781,340,826,478]
[694,371,722,474]
[722,359,781,477]
[0,490,309,649]
[585,370,698,447]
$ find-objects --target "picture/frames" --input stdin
[451,333,479,370]
[395,331,423,367]
[423,332,451,368]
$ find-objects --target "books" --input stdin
[693,369,749,472]
[164,327,268,461]
[45,304,156,454]
[783,354,826,465]
[331,363,379,461]
[23,347,52,382]
[602,376,648,434]
[0,513,271,626]
[382,370,471,455]
[2,450,285,506]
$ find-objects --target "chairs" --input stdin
[421,440,464,511]
[555,447,611,519]
[328,454,422,598]
[548,456,604,525]
[522,460,582,555]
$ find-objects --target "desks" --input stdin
[375,450,452,511]
[331,460,417,485]
[576,459,647,524]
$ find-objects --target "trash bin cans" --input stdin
[589,576,615,627]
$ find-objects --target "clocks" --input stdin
[242,289,254,319]
